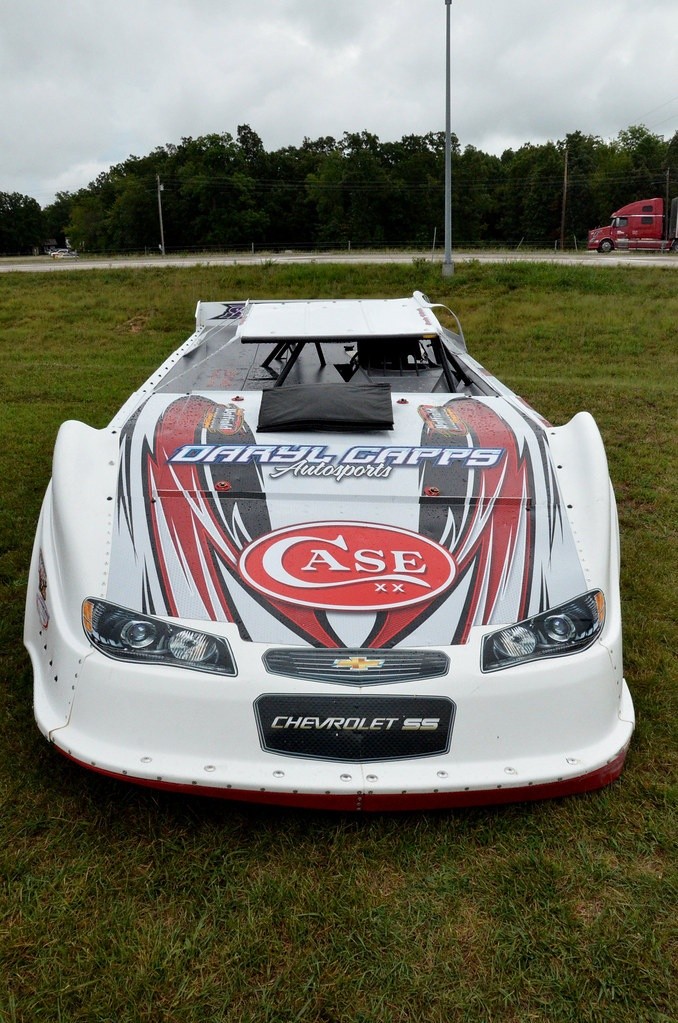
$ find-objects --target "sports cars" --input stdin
[26,292,638,809]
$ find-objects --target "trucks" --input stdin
[589,196,678,257]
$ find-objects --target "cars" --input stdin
[43,246,78,260]
